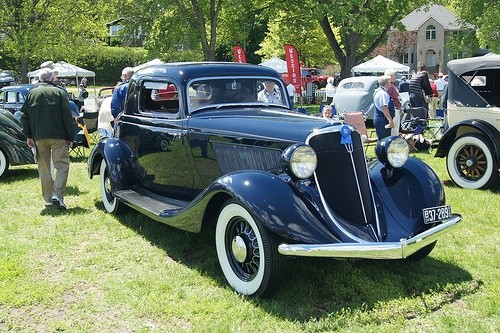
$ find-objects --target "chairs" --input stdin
[400,107,445,152]
[67,111,99,163]
[343,110,377,154]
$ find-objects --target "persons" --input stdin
[77,78,88,108]
[409,63,431,108]
[435,73,447,97]
[19,68,76,210]
[98,83,123,133]
[322,106,332,118]
[159,85,176,99]
[428,81,438,117]
[384,69,402,136]
[32,61,69,98]
[111,67,134,116]
[287,82,295,108]
[326,78,335,104]
[258,81,283,104]
[399,76,409,92]
[374,76,395,140]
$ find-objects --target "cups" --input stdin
[385,124,391,128]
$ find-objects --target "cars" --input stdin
[88,61,462,298]
[0,56,500,190]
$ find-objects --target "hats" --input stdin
[41,61,56,71]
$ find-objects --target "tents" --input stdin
[351,55,409,80]
[258,57,287,73]
[27,60,96,94]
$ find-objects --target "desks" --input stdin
[301,90,327,103]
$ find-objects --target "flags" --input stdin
[233,45,246,63]
[284,44,300,93]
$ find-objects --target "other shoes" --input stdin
[51,192,67,211]
[45,202,54,207]
